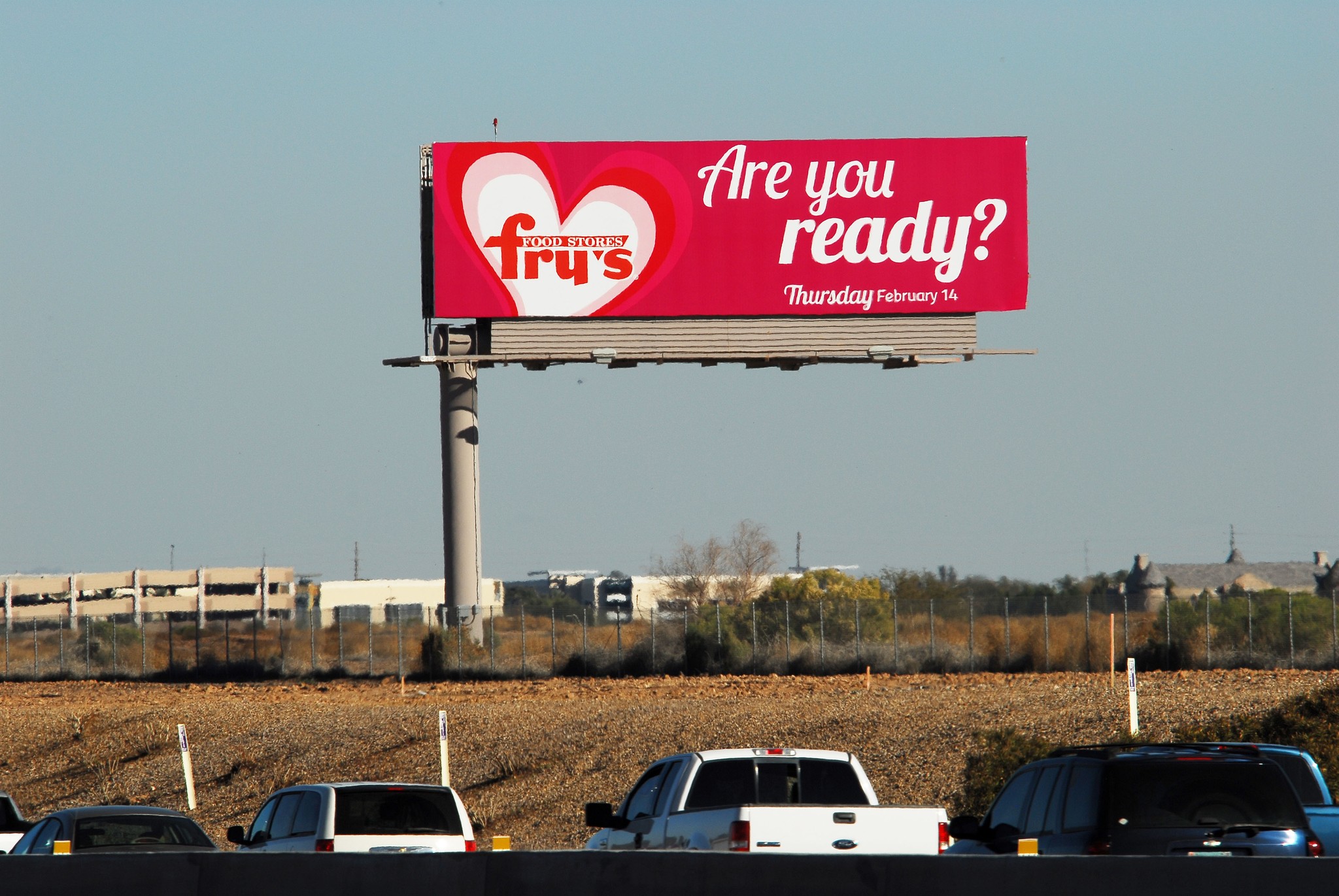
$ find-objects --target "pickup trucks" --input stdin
[581,747,955,853]
[1213,742,1339,858]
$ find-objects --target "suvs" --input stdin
[941,741,1323,857]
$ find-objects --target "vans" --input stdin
[226,782,475,853]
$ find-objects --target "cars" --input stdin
[6,804,224,856]
[0,790,25,855]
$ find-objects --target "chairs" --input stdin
[75,833,94,850]
[139,831,161,841]
